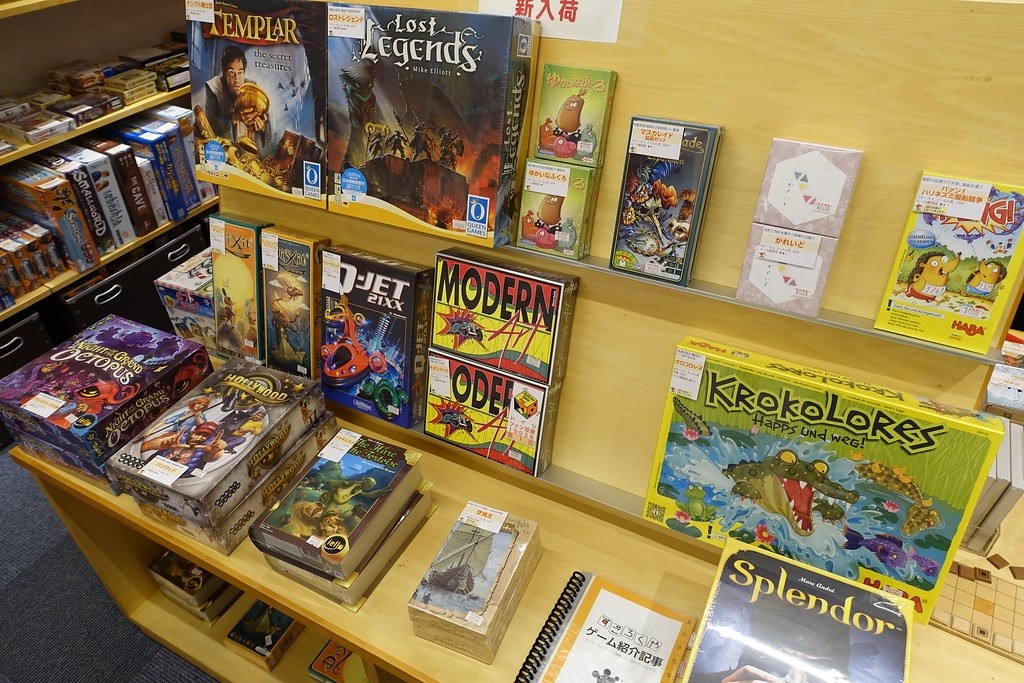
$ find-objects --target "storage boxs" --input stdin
[185,0,328,212]
[318,243,435,429]
[104,355,327,526]
[681,536,915,682]
[208,212,274,366]
[423,347,564,478]
[259,226,332,380]
[517,158,596,261]
[753,137,864,238]
[534,65,619,168]
[641,336,1006,627]
[223,598,305,672]
[0,26,218,496]
[734,222,839,319]
[327,3,541,249]
[430,248,580,387]
[132,412,341,556]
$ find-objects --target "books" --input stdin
[959,417,1023,552]
[513,570,697,683]
[147,426,438,683]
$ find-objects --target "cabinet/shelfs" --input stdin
[0,1,218,275]
[9,0,1024,683]
